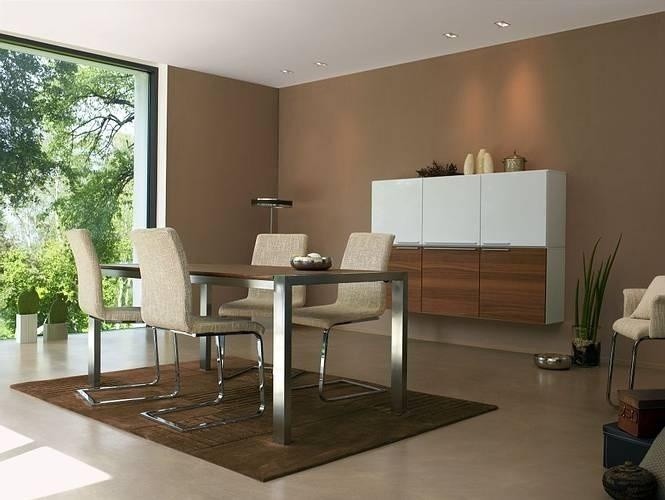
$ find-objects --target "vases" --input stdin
[463,149,494,175]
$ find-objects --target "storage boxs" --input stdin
[601,422,653,472]
[616,389,665,438]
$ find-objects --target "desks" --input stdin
[88,262,408,444]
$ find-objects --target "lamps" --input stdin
[251,198,293,234]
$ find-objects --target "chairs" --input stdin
[291,231,395,402]
[129,228,266,433]
[217,232,307,382]
[65,229,181,407]
[606,288,665,411]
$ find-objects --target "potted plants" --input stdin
[569,233,623,367]
[15,286,40,344]
[43,293,71,342]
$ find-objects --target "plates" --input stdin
[291,257,330,269]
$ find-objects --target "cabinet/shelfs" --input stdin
[370,178,422,314]
[422,169,567,326]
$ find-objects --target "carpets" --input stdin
[11,354,498,483]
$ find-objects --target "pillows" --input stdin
[630,275,665,320]
